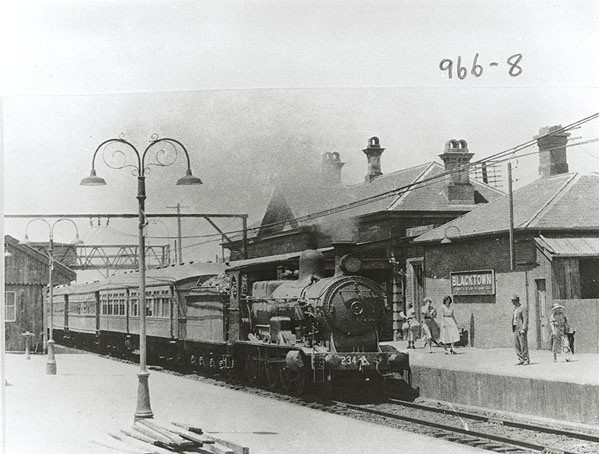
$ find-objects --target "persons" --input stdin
[511,293,530,365]
[549,303,570,364]
[420,298,440,353]
[438,295,459,355]
[401,302,416,348]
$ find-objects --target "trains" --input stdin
[42,238,423,403]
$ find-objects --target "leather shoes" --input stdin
[515,362,523,365]
[523,361,529,365]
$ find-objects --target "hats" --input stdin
[551,303,565,311]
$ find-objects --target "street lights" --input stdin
[21,217,85,378]
[78,129,204,428]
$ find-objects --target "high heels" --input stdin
[450,349,457,354]
[445,351,448,355]
